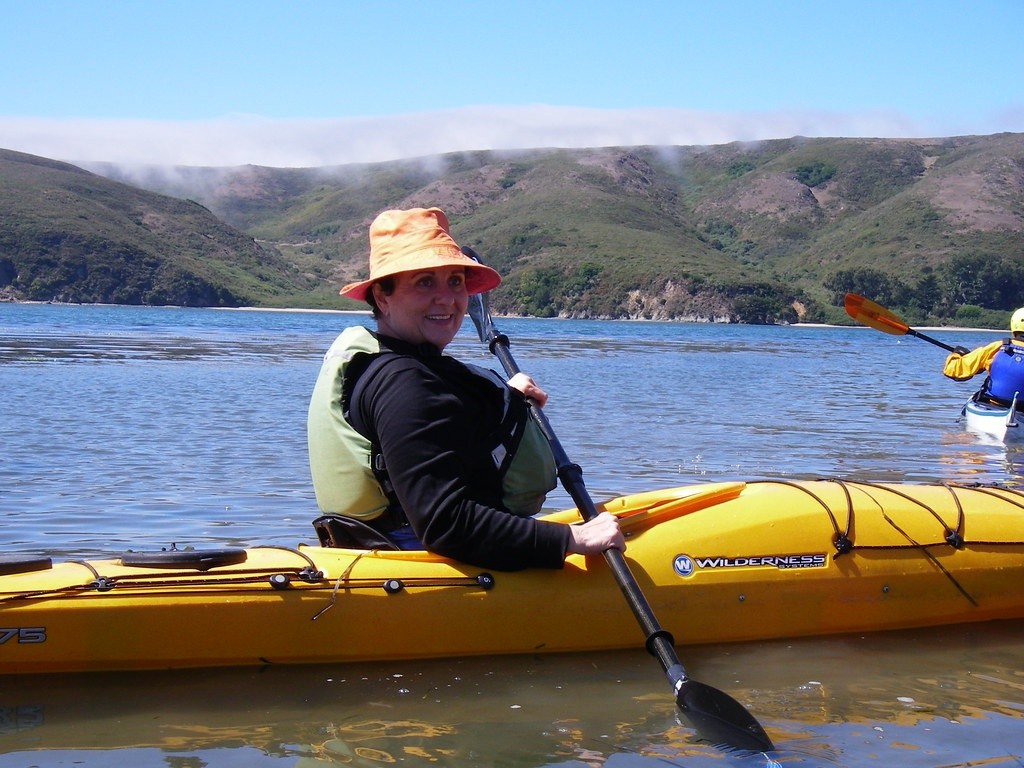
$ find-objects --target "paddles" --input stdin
[844,293,954,353]
[460,244,772,749]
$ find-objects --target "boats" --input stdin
[964,390,1024,441]
[1,473,1024,672]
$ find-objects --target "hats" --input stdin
[338,208,502,302]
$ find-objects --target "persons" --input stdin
[307,208,629,571]
[943,307,1024,411]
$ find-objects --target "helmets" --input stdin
[1011,308,1024,335]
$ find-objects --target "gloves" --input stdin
[955,345,970,356]
